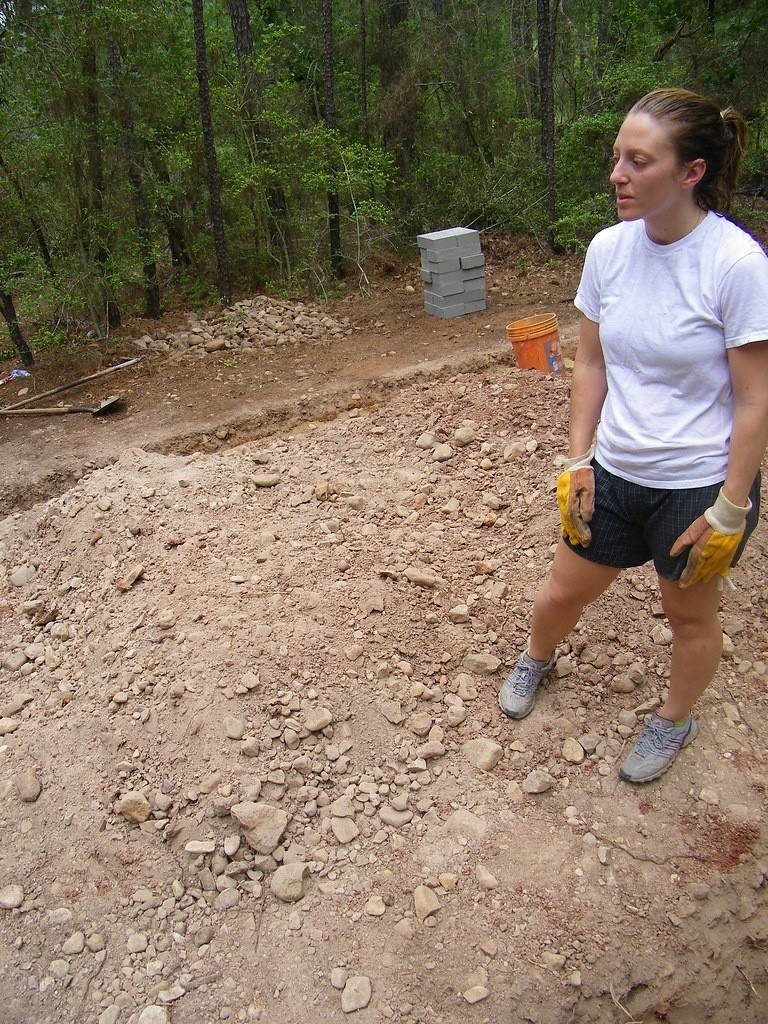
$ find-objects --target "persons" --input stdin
[498,87,767,785]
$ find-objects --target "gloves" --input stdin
[556,445,596,547]
[670,486,753,591]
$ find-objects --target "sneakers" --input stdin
[620,707,698,783]
[498,645,557,720]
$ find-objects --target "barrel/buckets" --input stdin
[506,313,566,377]
